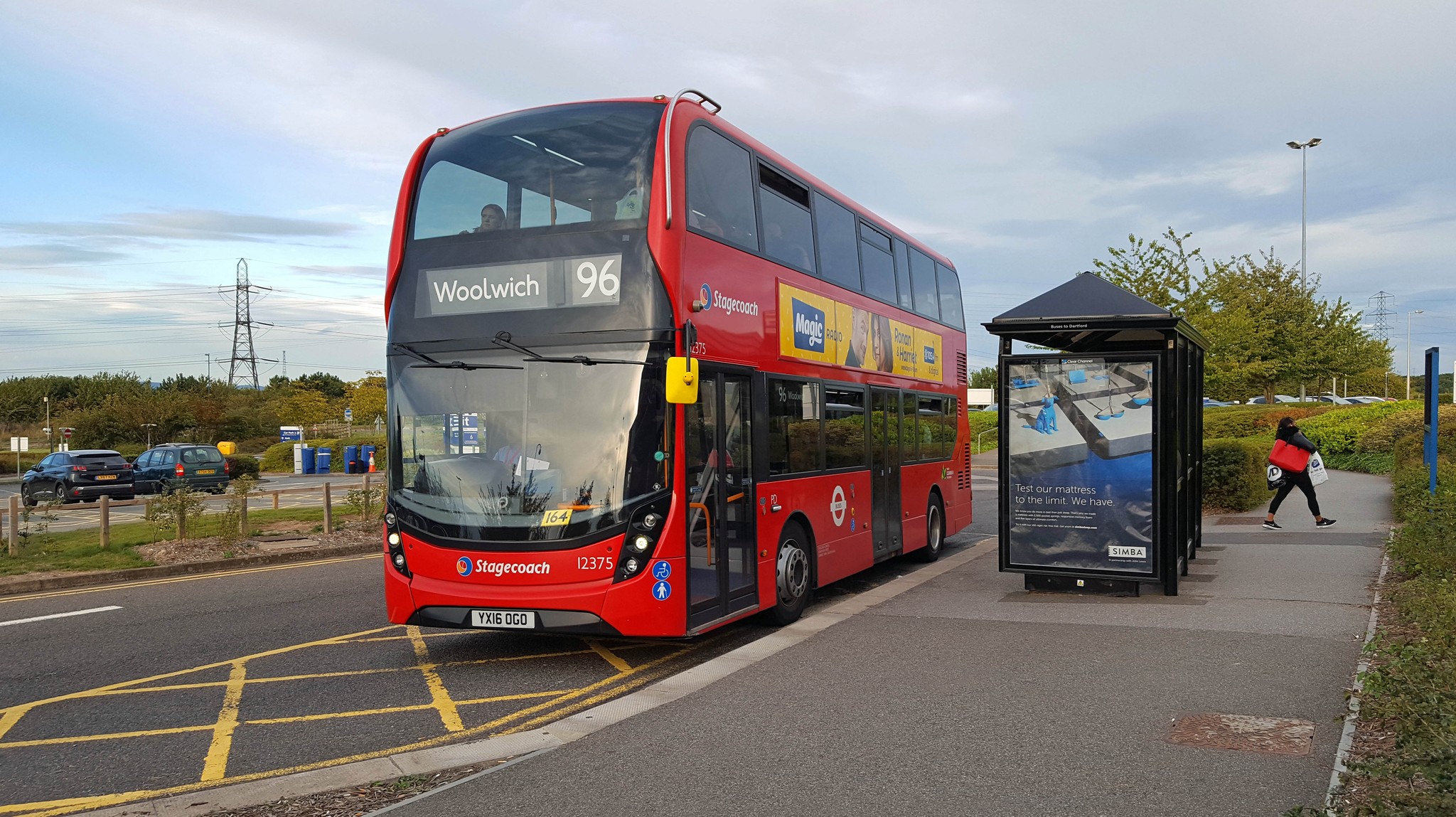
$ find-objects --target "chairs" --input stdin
[171,454,206,461]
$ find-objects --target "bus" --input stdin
[381,90,974,640]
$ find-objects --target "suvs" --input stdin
[20,450,135,512]
[132,444,229,496]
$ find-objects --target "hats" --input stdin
[1280,417,1294,428]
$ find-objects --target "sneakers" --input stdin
[1316,517,1337,528]
[1263,519,1282,530]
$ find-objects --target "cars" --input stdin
[1201,397,1241,406]
[1244,395,1397,407]
[968,403,999,412]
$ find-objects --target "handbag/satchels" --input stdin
[614,188,644,220]
[1308,451,1329,487]
[1267,463,1287,491]
[1269,439,1311,473]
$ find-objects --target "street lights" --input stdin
[204,353,210,395]
[1287,137,1324,306]
[1406,309,1422,401]
[140,423,156,450]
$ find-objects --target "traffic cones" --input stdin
[368,449,377,474]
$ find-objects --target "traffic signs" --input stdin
[442,412,488,447]
[280,426,304,444]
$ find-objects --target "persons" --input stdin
[845,307,869,368]
[493,427,522,481]
[459,204,507,235]
[703,212,725,238]
[871,313,893,373]
[791,451,810,471]
[1262,417,1337,529]
[614,187,642,220]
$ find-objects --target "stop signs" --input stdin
[65,429,72,438]
[344,410,352,421]
